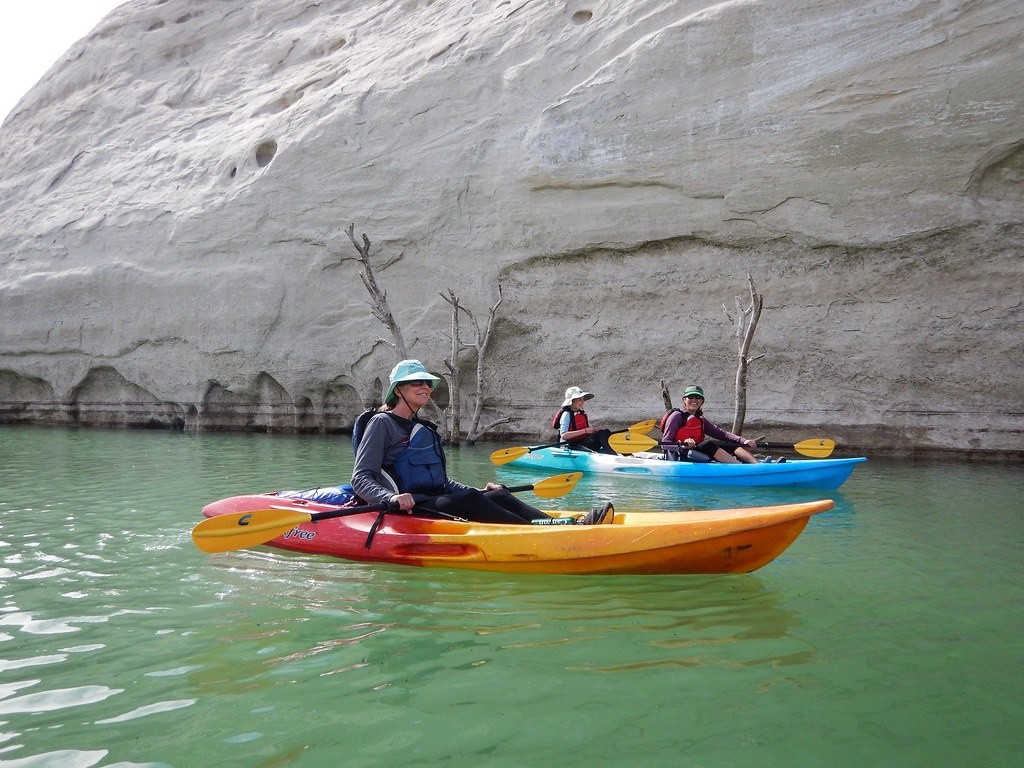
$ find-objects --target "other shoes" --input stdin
[761,456,771,463]
[577,501,614,524]
[775,457,786,463]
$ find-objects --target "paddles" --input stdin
[607,430,837,461]
[489,417,656,467]
[191,470,583,556]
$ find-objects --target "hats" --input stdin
[561,387,594,408]
[683,386,704,400]
[385,359,442,408]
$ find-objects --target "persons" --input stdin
[559,386,634,457]
[662,385,760,464]
[350,360,615,526]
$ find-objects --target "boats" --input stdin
[507,441,868,491]
[197,491,837,579]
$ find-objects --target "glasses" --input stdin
[687,395,700,400]
[397,379,433,388]
